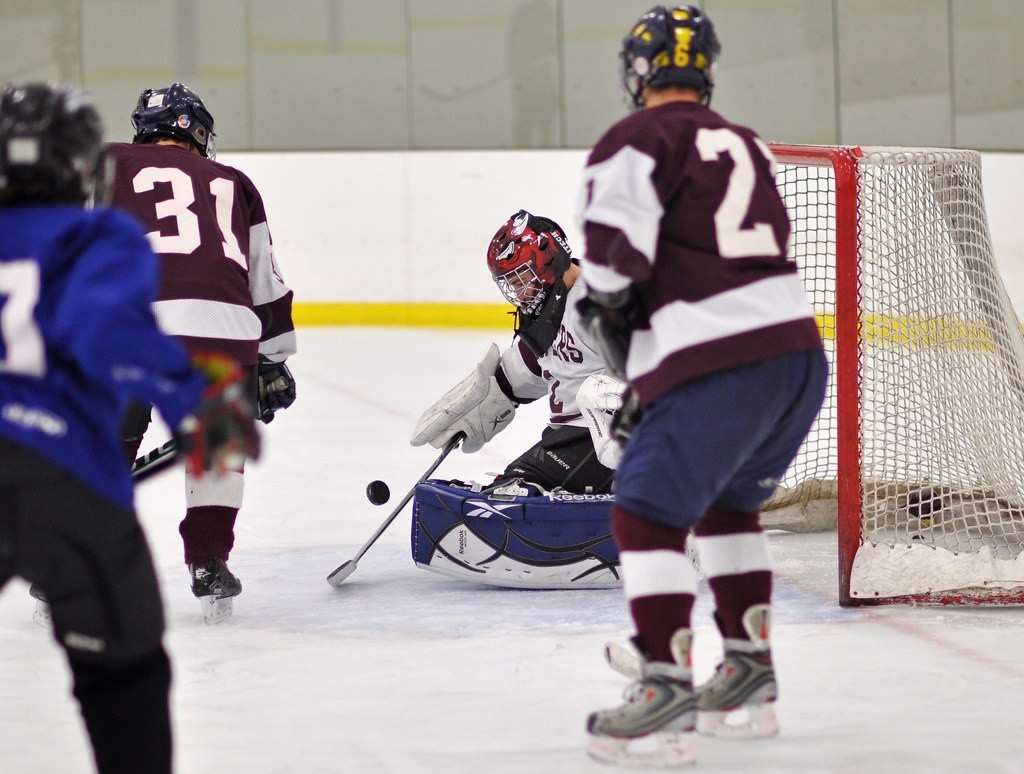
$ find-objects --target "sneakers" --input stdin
[29,581,54,629]
[584,628,699,767]
[188,557,242,625]
[692,604,781,738]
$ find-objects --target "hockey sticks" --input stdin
[131,374,290,480]
[325,432,466,586]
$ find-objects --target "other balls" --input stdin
[366,480,390,505]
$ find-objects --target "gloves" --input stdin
[176,376,261,479]
[255,352,296,425]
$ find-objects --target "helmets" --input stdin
[488,210,572,317]
[131,83,219,161]
[616,5,721,111]
[0,81,102,207]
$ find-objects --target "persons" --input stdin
[410,208,705,594]
[0,78,265,774]
[29,82,297,627]
[586,6,828,767]
